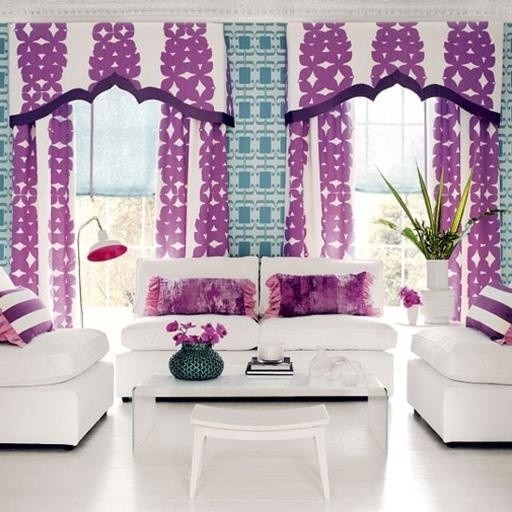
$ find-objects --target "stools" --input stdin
[189,403,331,499]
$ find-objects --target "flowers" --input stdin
[166,320,227,346]
[398,286,423,308]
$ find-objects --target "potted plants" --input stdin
[371,156,512,324]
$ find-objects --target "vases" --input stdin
[169,342,223,380]
[407,305,419,325]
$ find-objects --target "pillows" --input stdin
[263,272,378,318]
[465,278,512,344]
[146,275,256,314]
[0,285,56,345]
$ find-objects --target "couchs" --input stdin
[114,255,396,403]
[1,265,114,451]
[406,282,512,448]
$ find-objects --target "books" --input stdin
[244,361,294,375]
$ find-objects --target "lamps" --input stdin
[77,216,128,329]
